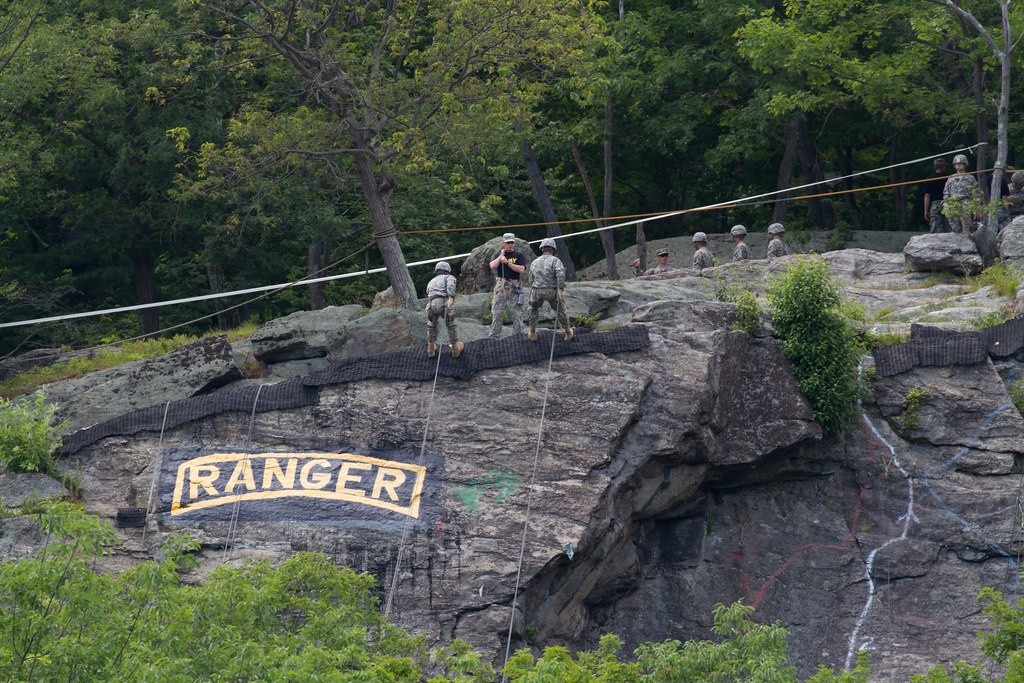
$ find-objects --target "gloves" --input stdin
[445,297,456,311]
[557,289,564,301]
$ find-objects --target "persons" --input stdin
[924,158,950,234]
[528,239,574,342]
[692,232,712,269]
[489,232,525,340]
[634,249,673,276]
[426,262,464,358]
[766,223,788,258]
[731,225,753,261]
[987,161,1024,222]
[943,154,978,233]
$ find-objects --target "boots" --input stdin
[528,327,538,341]
[428,343,437,357]
[452,342,464,357]
[563,327,573,341]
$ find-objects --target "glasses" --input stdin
[659,254,669,257]
[507,242,514,244]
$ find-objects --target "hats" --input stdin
[503,233,516,243]
[934,158,948,167]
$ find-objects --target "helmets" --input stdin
[435,261,452,272]
[656,248,669,255]
[731,224,747,236]
[539,238,557,251]
[693,232,708,243]
[1011,171,1024,185]
[768,223,786,234]
[953,154,969,167]
